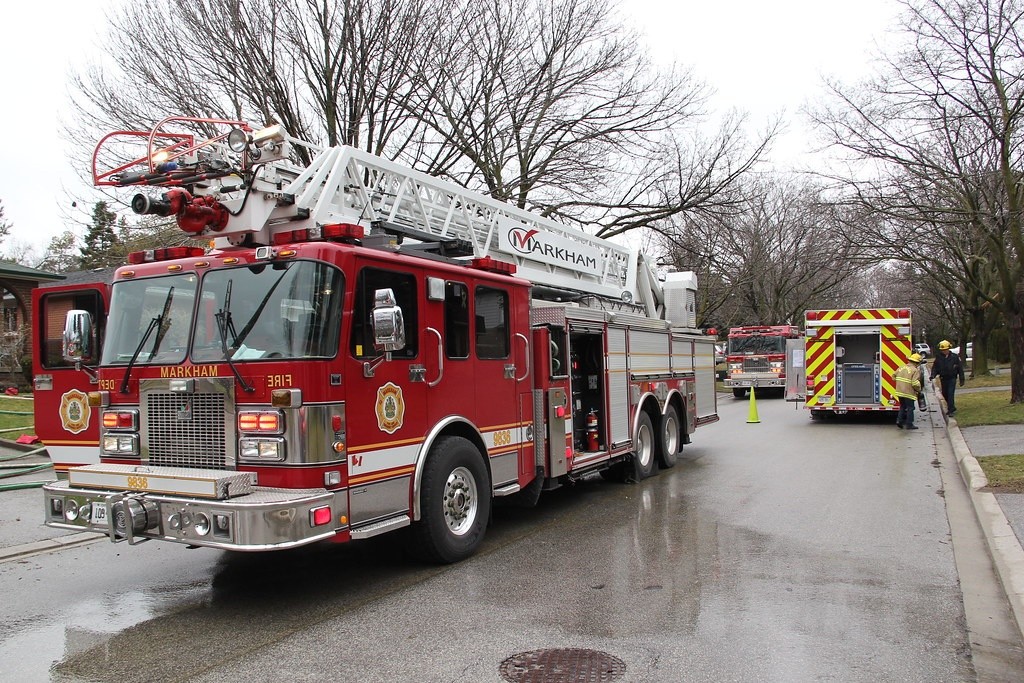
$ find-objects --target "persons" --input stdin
[892,353,923,430]
[928,339,965,415]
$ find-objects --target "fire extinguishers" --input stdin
[584,408,599,453]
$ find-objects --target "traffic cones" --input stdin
[746,387,761,423]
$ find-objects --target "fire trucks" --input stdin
[785,308,916,419]
[723,325,806,397]
[32,117,720,566]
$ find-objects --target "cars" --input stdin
[715,345,728,363]
[949,342,973,359]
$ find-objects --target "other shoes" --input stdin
[946,408,957,415]
[897,421,903,429]
[906,423,918,429]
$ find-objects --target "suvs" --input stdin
[915,343,931,359]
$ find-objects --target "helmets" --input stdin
[938,340,950,350]
[908,353,923,363]
